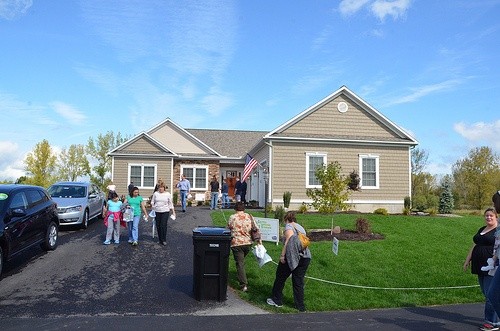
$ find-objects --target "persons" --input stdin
[102,193,125,244]
[225,203,262,291]
[222,179,230,208]
[150,183,175,244]
[463,207,500,330]
[479,189,500,322]
[267,210,311,312]
[128,181,135,194]
[240,180,247,200]
[121,187,149,245]
[234,176,242,204]
[210,175,219,210]
[176,174,190,213]
[152,177,168,194]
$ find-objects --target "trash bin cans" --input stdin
[191,226,231,303]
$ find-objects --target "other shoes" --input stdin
[128,240,132,243]
[132,241,138,245]
[239,285,247,292]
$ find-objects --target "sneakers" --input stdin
[480,323,499,331]
[266,298,283,307]
[114,240,119,244]
[103,240,111,244]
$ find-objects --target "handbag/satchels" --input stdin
[292,223,310,251]
[122,207,134,222]
[250,214,261,241]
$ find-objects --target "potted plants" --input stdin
[283,191,292,213]
[274,205,286,238]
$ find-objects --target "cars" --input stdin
[43,182,108,228]
[0,182,60,279]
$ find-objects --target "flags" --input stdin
[242,153,258,183]
